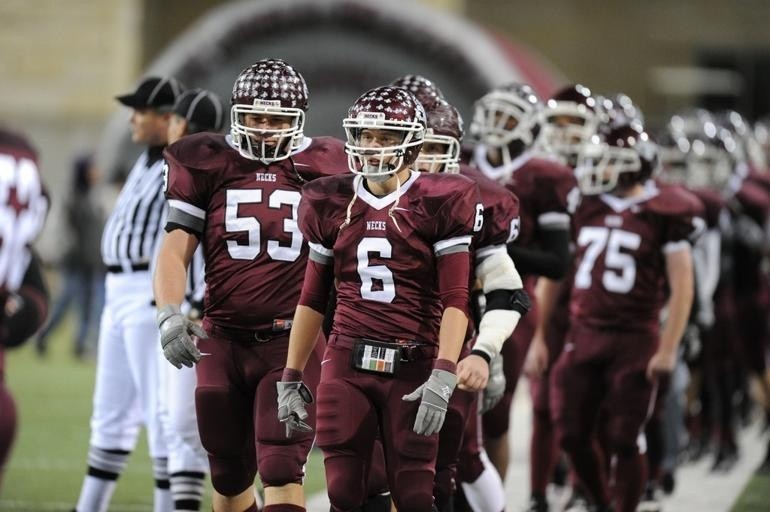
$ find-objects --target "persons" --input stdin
[35,154,108,357]
[68,56,769,510]
[0,129,55,484]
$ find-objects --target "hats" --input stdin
[157,87,224,132]
[118,77,186,109]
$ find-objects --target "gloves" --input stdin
[402,368,458,437]
[479,358,507,414]
[156,304,207,368]
[276,380,314,438]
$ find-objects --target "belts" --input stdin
[329,334,439,359]
[200,316,290,342]
[108,263,148,273]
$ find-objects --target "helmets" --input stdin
[417,97,465,173]
[545,83,598,150]
[342,85,427,181]
[387,75,442,111]
[230,57,309,164]
[575,124,659,195]
[469,84,541,150]
